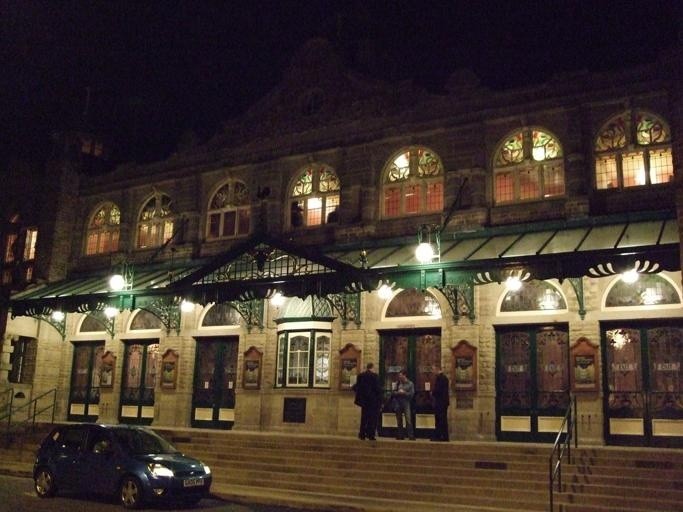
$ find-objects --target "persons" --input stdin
[392,369,417,441]
[291,201,339,227]
[354,362,384,440]
[428,363,450,441]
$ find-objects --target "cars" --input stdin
[34,423,212,509]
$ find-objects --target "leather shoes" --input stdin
[358,432,376,440]
